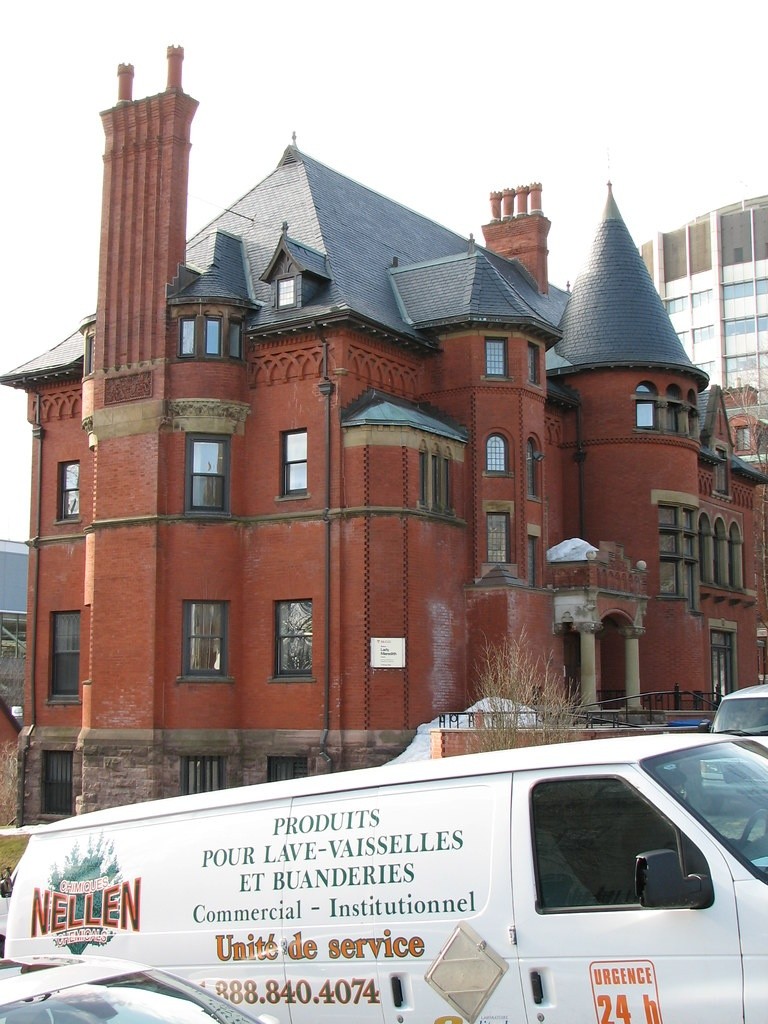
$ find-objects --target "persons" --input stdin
[3,866,12,894]
[604,768,768,913]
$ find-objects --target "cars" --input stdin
[1,952,268,1024]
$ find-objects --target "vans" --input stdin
[695,679,768,819]
[3,730,768,1024]
